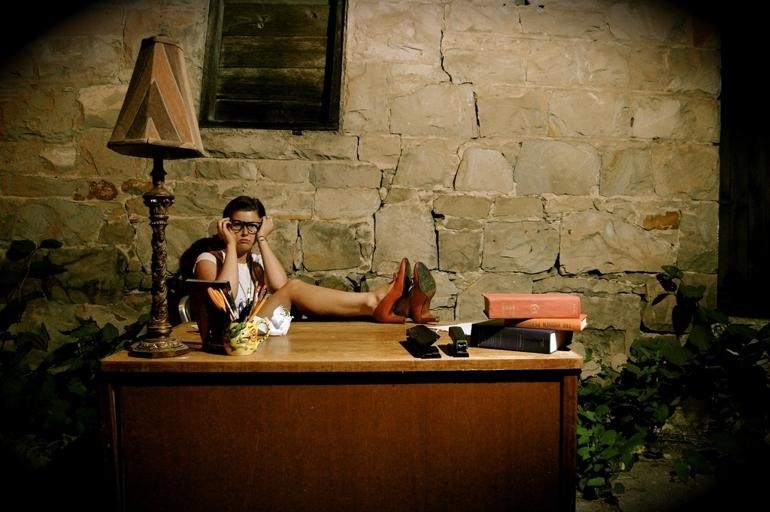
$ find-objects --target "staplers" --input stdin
[447,327,469,357]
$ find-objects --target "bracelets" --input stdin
[257,236,268,242]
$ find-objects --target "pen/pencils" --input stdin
[219,280,270,322]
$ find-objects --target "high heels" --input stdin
[371,255,440,323]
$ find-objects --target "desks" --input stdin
[101,318,583,511]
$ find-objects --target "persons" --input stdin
[192,196,441,326]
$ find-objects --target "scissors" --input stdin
[207,287,228,314]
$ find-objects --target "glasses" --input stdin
[230,219,262,233]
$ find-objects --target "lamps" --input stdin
[110,35,205,358]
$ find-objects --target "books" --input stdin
[469,294,588,353]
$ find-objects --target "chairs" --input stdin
[177,236,229,324]
